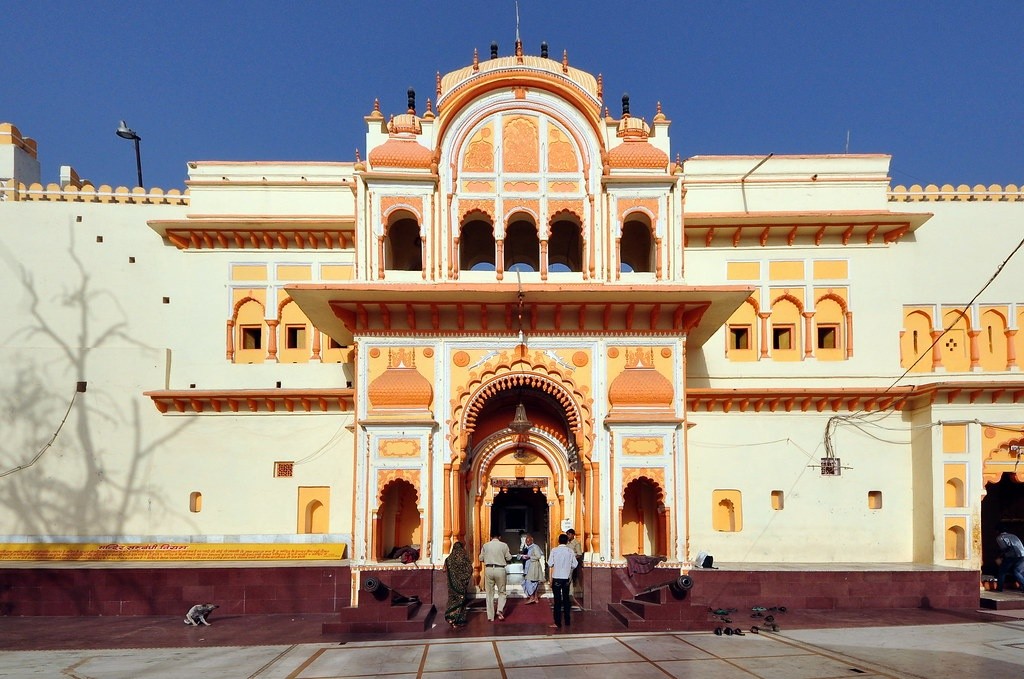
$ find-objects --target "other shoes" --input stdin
[715,627,722,635]
[773,624,780,632]
[759,622,773,631]
[724,628,732,635]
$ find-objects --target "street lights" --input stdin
[114,121,143,187]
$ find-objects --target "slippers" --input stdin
[750,626,759,634]
[733,628,745,636]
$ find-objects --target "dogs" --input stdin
[184,603,219,626]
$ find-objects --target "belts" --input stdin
[486,564,504,568]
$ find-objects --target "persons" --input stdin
[516,535,541,605]
[444,542,473,629]
[994,527,1024,593]
[479,530,512,622]
[548,534,578,627]
[562,530,582,585]
